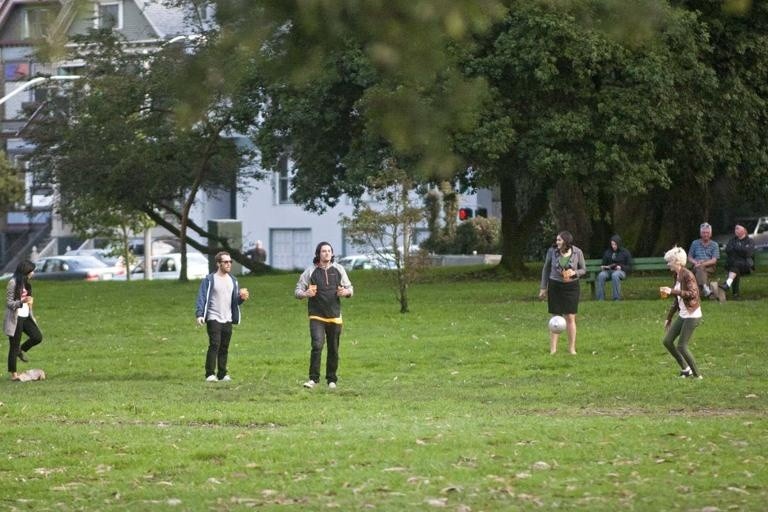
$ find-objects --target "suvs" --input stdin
[714,216,767,254]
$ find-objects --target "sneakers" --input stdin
[10,374,18,380]
[329,382,336,388]
[304,380,315,387]
[679,369,692,379]
[18,350,28,361]
[207,375,217,382]
[222,375,230,380]
[703,287,713,297]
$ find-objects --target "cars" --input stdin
[31,255,126,282]
[112,253,209,281]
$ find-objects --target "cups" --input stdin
[563,271,570,280]
[660,287,668,298]
[310,285,316,296]
[28,297,33,306]
[240,288,247,299]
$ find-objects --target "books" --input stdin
[600,262,618,271]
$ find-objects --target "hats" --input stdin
[737,220,751,234]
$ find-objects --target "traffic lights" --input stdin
[459,206,472,220]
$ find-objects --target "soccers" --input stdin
[548,316,566,334]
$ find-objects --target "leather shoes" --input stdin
[719,281,729,291]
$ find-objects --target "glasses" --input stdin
[218,260,233,264]
[701,223,709,227]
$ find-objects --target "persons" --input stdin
[718,221,755,298]
[597,233,633,301]
[194,251,249,383]
[688,222,720,296]
[4,259,44,381]
[246,239,267,264]
[294,241,354,389]
[659,246,703,380]
[538,230,587,355]
